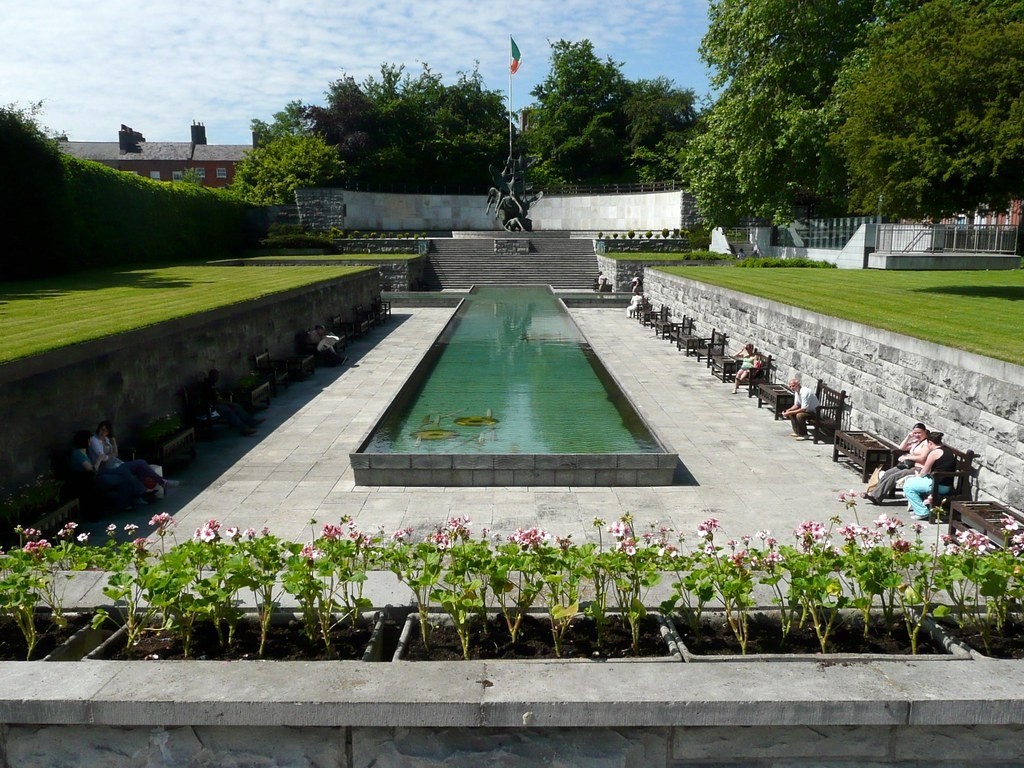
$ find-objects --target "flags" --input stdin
[509,38,523,74]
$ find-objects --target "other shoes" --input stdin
[910,511,929,520]
[860,492,882,505]
[791,432,809,441]
[243,428,257,436]
[250,418,265,427]
[124,481,167,509]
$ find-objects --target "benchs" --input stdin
[732,347,775,395]
[890,439,974,517]
[355,304,376,330]
[696,329,727,367]
[374,295,391,318]
[649,303,670,330]
[670,315,694,348]
[633,297,647,319]
[185,376,234,442]
[255,349,290,395]
[330,315,356,341]
[301,327,332,362]
[795,379,847,442]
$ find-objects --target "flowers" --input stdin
[140,412,183,445]
[2,472,66,527]
[235,372,261,393]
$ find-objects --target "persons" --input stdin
[631,272,640,292]
[306,324,349,365]
[374,297,381,324]
[733,343,759,395]
[781,379,824,441]
[597,272,608,292]
[71,421,182,507]
[626,292,643,318]
[183,367,264,438]
[902,431,956,523]
[862,422,929,504]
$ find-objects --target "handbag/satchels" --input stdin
[897,459,915,469]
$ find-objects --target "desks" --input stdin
[641,310,652,323]
[236,379,269,408]
[656,320,670,338]
[711,355,738,383]
[375,309,386,321]
[330,333,348,354]
[679,334,704,357]
[834,427,899,484]
[353,318,369,336]
[17,496,82,549]
[758,382,795,421]
[288,353,314,380]
[944,499,1024,558]
[155,425,196,471]
